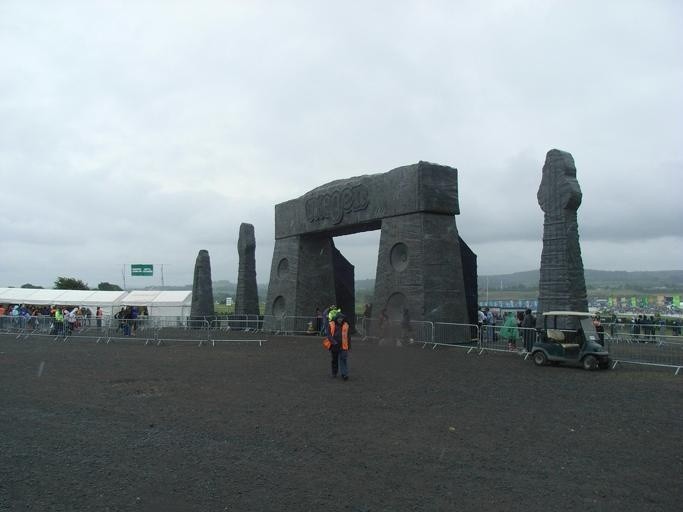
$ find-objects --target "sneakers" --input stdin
[332,371,337,377]
[343,375,348,379]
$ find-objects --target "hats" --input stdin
[334,312,347,321]
[329,305,337,310]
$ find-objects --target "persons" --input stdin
[315,303,412,347]
[594,311,681,344]
[478,305,536,352]
[322,313,351,379]
[0,303,148,339]
